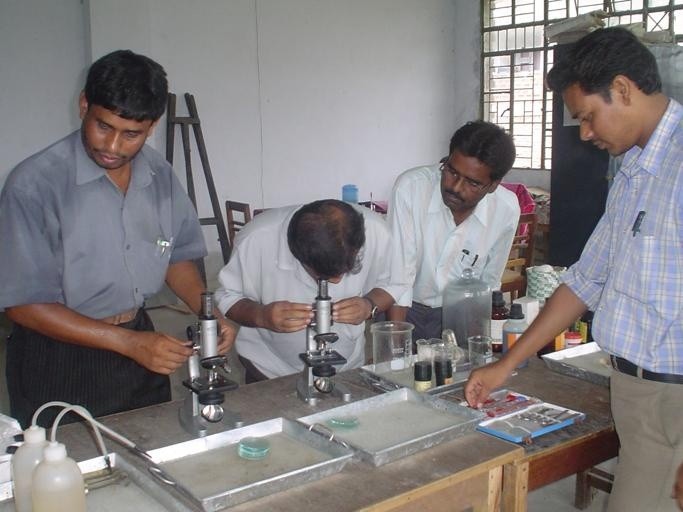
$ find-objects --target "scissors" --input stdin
[521,408,569,427]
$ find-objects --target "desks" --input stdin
[0,342,619,511]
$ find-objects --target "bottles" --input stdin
[414,268,594,387]
[11,425,87,512]
[342,183,359,205]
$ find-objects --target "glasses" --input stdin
[438,153,496,198]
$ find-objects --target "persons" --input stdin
[1,49,235,430]
[215,199,401,383]
[462,26,683,512]
[390,120,521,355]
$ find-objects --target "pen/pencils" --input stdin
[369,192,373,211]
[632,211,645,236]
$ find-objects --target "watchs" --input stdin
[364,296,380,322]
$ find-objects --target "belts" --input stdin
[610,354,683,384]
[101,308,139,326]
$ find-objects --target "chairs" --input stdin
[501,212,538,298]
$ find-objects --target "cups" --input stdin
[369,320,414,387]
